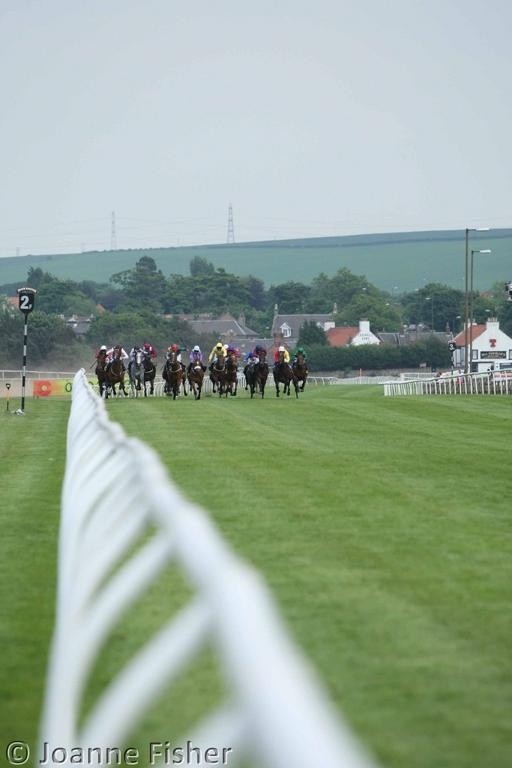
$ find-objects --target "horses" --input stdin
[93,351,269,400]
[272,360,299,400]
[292,353,308,393]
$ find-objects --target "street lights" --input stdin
[361,282,491,335]
[469,246,492,372]
[465,226,491,370]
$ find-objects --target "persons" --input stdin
[95,342,309,381]
